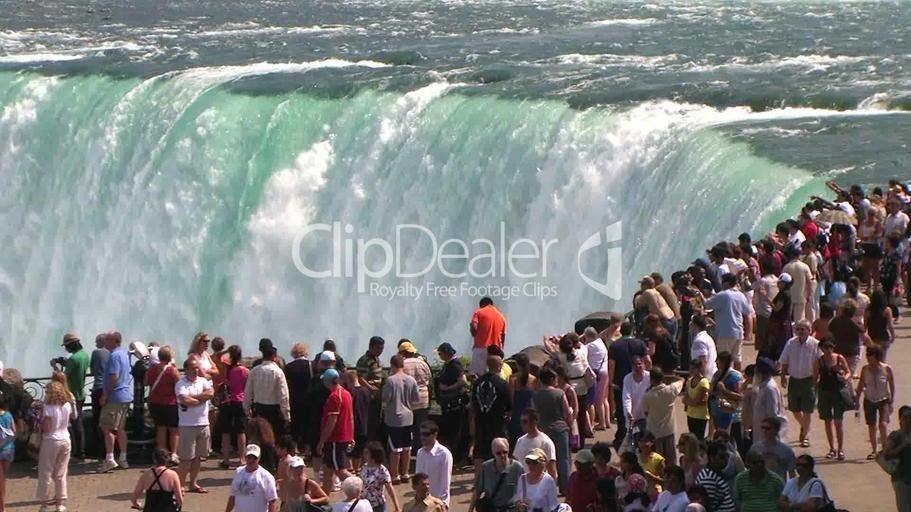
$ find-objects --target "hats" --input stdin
[638,271,664,289]
[524,448,547,463]
[399,341,416,353]
[288,456,305,469]
[573,449,594,465]
[775,273,794,282]
[691,258,710,267]
[820,336,836,348]
[245,443,260,457]
[319,368,339,382]
[319,350,335,363]
[628,473,647,492]
[60,333,80,347]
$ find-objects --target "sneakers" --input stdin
[390,474,410,484]
[97,459,129,474]
[170,454,181,466]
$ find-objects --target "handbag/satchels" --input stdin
[210,383,230,406]
[307,502,333,511]
[840,382,857,411]
[0,425,16,449]
[476,497,498,511]
[584,367,598,391]
[876,450,900,476]
[818,501,836,512]
[716,393,739,414]
[440,395,472,420]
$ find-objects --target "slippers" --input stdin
[586,422,612,438]
[221,462,230,469]
[188,486,207,492]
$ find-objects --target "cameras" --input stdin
[548,336,554,340]
[54,357,65,367]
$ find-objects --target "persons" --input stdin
[30,371,78,472]
[0,392,16,512]
[30,379,74,512]
[0,368,40,471]
[87,183,911,512]
[49,331,89,460]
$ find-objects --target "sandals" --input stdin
[826,450,844,460]
[799,427,810,447]
[868,452,876,459]
